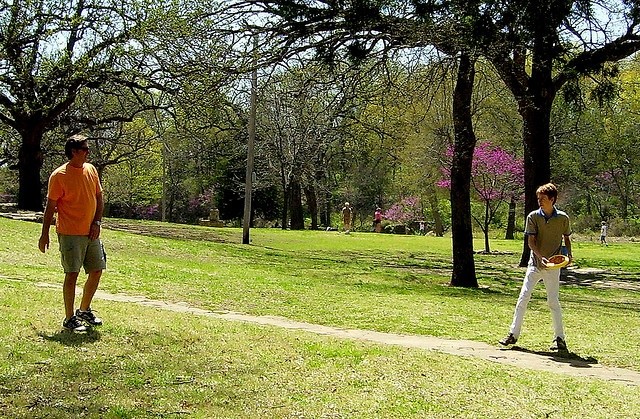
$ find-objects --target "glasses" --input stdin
[77,146,90,151]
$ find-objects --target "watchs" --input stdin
[93,220,102,226]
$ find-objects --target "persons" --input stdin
[419,221,425,236]
[498,182,572,350]
[374,208,382,232]
[38,134,106,332]
[343,202,351,234]
[601,221,609,247]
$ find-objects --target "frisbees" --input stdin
[545,255,569,270]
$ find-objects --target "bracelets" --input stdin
[568,252,572,258]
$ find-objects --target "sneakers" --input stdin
[63,315,89,333]
[75,307,102,326]
[550,336,567,349]
[498,333,518,345]
